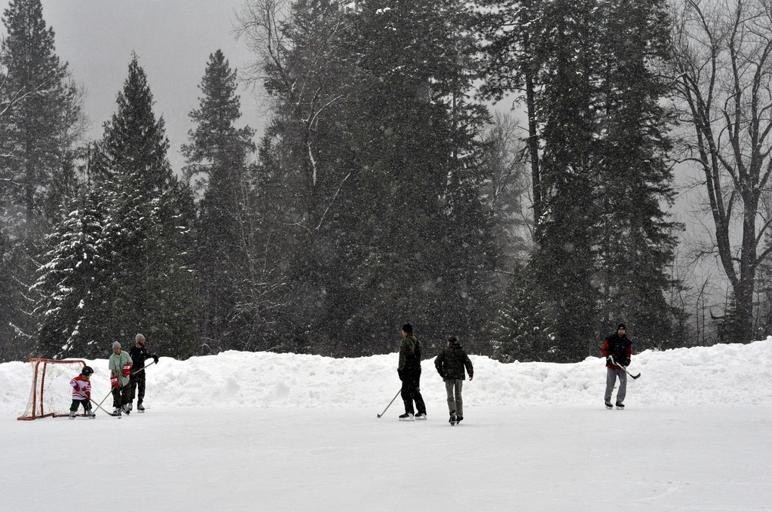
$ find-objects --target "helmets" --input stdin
[82,366,94,375]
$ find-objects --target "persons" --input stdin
[69,366,96,417]
[108,341,134,414]
[396,323,427,419]
[434,337,474,422]
[598,323,633,407]
[128,333,159,410]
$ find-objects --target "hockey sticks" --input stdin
[91,387,115,417]
[616,362,640,379]
[90,399,121,416]
[377,391,401,417]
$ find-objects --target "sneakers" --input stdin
[399,410,427,418]
[449,416,463,423]
[605,400,624,407]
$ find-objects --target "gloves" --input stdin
[110,376,120,390]
[73,381,80,391]
[152,355,158,364]
[85,391,90,400]
[123,364,130,377]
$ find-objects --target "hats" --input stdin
[618,324,625,329]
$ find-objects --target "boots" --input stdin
[69,410,75,417]
[113,402,145,414]
[88,410,95,417]
